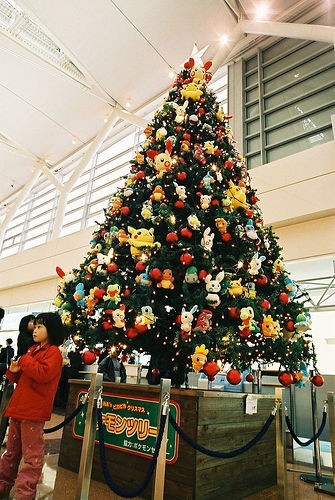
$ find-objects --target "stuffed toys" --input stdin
[53,43,310,388]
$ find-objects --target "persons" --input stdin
[0,312,62,500]
[1,307,168,424]
